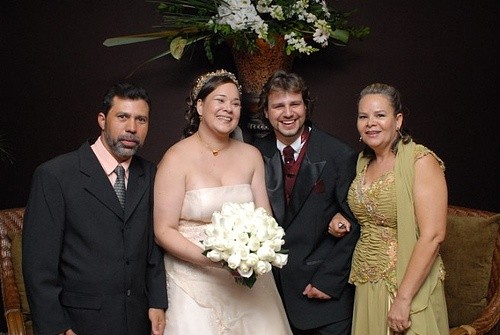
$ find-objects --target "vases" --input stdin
[226,36,293,113]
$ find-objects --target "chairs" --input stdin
[437,206,500,335]
[0,208,35,335]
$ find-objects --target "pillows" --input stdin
[8,231,31,314]
[439,214,500,327]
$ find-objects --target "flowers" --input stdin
[199,202,290,288]
[102,0,371,80]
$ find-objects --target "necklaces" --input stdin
[201,138,229,156]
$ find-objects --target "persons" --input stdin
[154,70,291,335]
[252,70,361,335]
[22,84,168,335]
[328,84,449,335]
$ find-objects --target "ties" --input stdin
[281,146,297,164]
[112,165,127,209]
